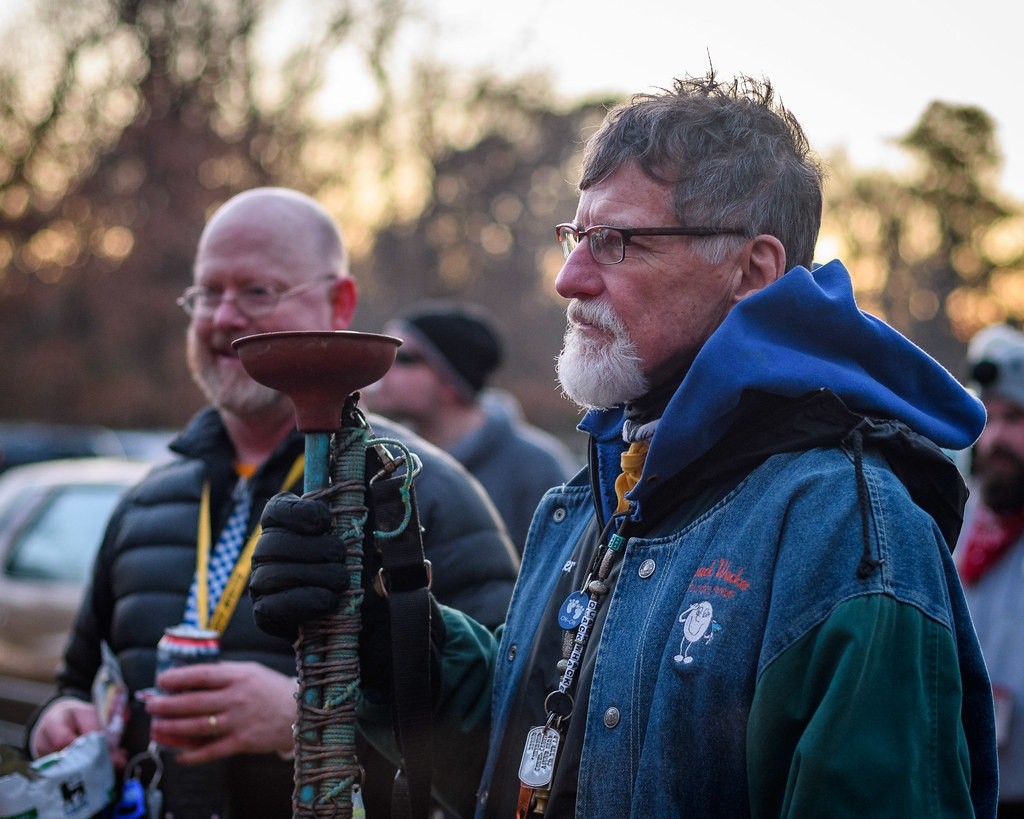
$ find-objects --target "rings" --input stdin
[209,715,217,736]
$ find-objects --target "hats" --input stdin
[389,309,500,400]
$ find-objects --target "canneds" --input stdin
[150,624,223,751]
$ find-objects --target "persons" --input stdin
[248,76,1000,819]
[367,310,578,560]
[952,323,1024,819]
[25,187,520,818]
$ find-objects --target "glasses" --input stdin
[555,223,750,265]
[179,273,336,322]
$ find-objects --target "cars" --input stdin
[0,457,169,784]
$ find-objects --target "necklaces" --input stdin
[516,512,632,818]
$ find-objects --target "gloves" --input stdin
[247,489,384,650]
